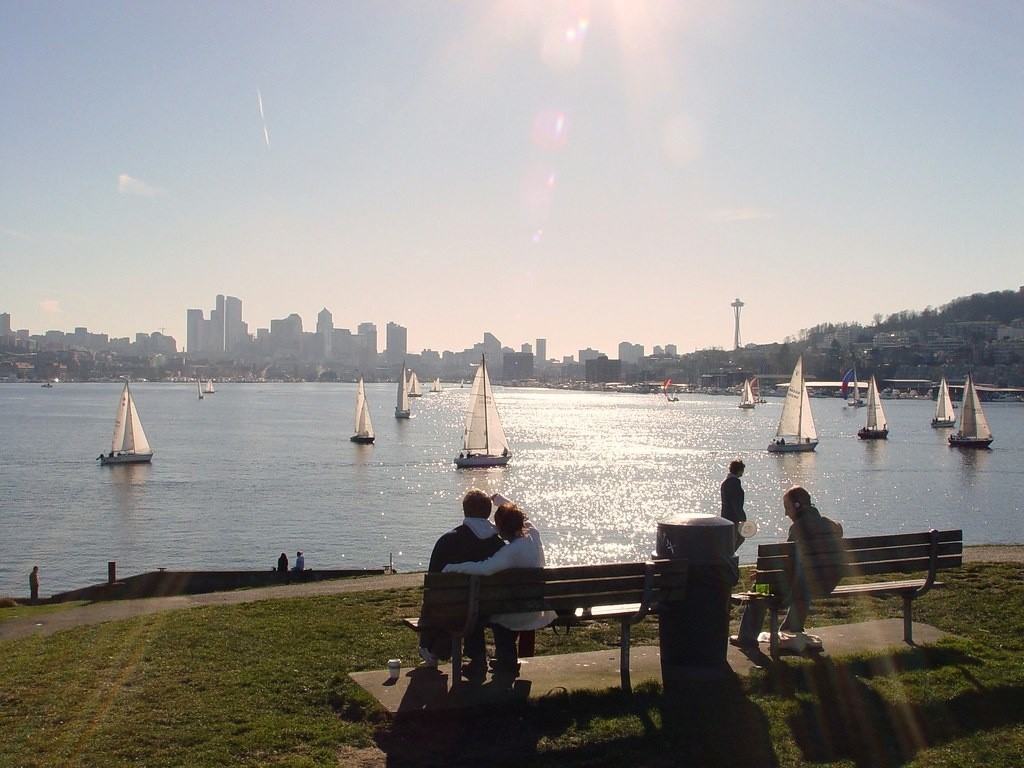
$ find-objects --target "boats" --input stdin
[806,387,867,399]
[40,383,52,387]
[992,395,1018,402]
[707,387,788,397]
[879,387,933,400]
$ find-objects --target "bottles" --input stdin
[756,570,769,596]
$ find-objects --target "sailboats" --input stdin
[664,378,679,401]
[394,360,410,418]
[429,377,442,392]
[95,380,153,465]
[407,371,423,397]
[349,371,375,443]
[461,379,464,388]
[766,352,819,452]
[738,377,768,408]
[453,352,513,467]
[947,372,994,448]
[203,379,215,393]
[857,373,889,439]
[930,379,957,427]
[841,361,865,407]
[198,379,204,399]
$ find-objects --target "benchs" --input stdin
[732,526,965,656]
[400,551,672,696]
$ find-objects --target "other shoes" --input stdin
[489,660,521,672]
[462,662,488,674]
[730,635,759,648]
[418,646,438,667]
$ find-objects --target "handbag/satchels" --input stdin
[550,607,577,637]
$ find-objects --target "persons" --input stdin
[460,452,464,458]
[721,461,746,553]
[419,491,506,669]
[29,566,39,599]
[467,452,474,459]
[776,437,785,445]
[278,552,289,571]
[291,551,304,570]
[442,493,559,677]
[729,486,844,647]
[117,452,122,456]
[109,451,114,457]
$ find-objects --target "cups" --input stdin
[388,659,401,678]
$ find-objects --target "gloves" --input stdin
[441,563,465,574]
[491,493,498,501]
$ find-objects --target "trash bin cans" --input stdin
[651,513,738,692]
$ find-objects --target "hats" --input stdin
[738,520,757,538]
